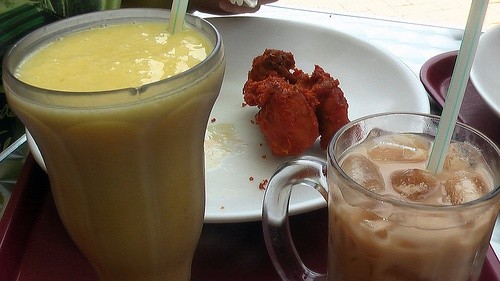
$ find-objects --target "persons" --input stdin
[121,0,279,15]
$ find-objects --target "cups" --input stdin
[262,112,500,281]
[2,8,226,281]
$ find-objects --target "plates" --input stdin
[24,17,430,223]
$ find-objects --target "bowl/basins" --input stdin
[469,24,500,116]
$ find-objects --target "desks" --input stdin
[0,3,500,261]
